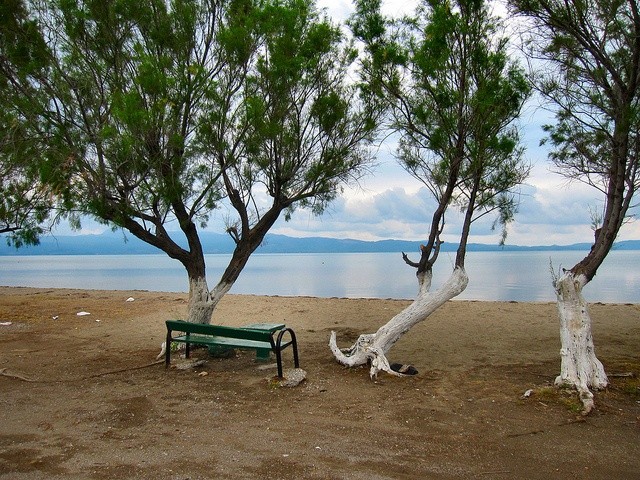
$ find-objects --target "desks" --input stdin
[240,323,286,362]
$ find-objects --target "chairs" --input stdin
[165,318,299,379]
